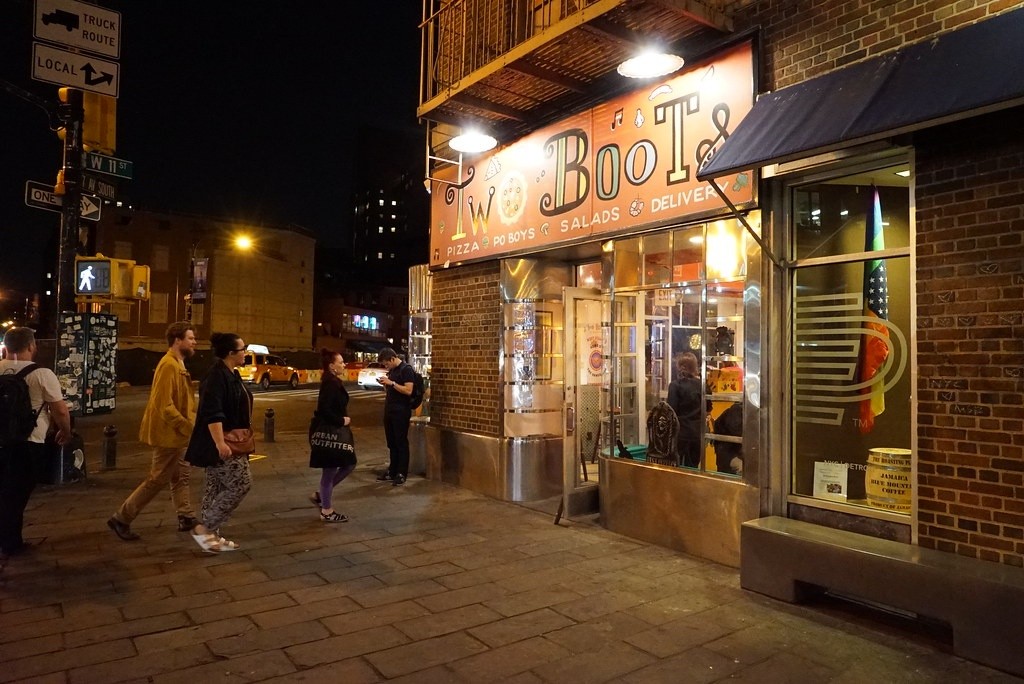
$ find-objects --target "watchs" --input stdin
[392,382,395,386]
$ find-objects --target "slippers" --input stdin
[189,528,220,554]
[201,536,240,551]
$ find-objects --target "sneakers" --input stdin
[108,515,140,540]
[177,516,201,532]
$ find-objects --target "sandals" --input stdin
[310,490,322,509]
[320,509,350,523]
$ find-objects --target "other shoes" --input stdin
[2,540,27,554]
[377,473,393,480]
[393,474,406,486]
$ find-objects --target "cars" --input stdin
[358,362,390,392]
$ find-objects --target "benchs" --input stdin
[742,515,1024,679]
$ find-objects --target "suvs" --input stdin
[235,343,300,392]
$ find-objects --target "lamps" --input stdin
[616,49,684,78]
[449,123,502,154]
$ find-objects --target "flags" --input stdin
[853,186,890,433]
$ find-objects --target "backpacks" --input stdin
[398,363,423,410]
[0,363,46,444]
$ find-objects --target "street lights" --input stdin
[187,231,254,321]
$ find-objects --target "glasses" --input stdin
[234,346,248,352]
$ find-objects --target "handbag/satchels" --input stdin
[222,428,255,455]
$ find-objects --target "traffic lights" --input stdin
[74,255,113,295]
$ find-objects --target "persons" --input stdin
[667,352,713,468]
[0,327,73,555]
[376,348,415,486]
[106,322,204,541]
[713,402,743,476]
[184,332,254,554]
[309,352,357,522]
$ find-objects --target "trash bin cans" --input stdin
[407,416,430,474]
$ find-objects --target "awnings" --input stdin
[696,8,1024,266]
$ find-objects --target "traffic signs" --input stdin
[30,41,120,100]
[25,180,102,223]
